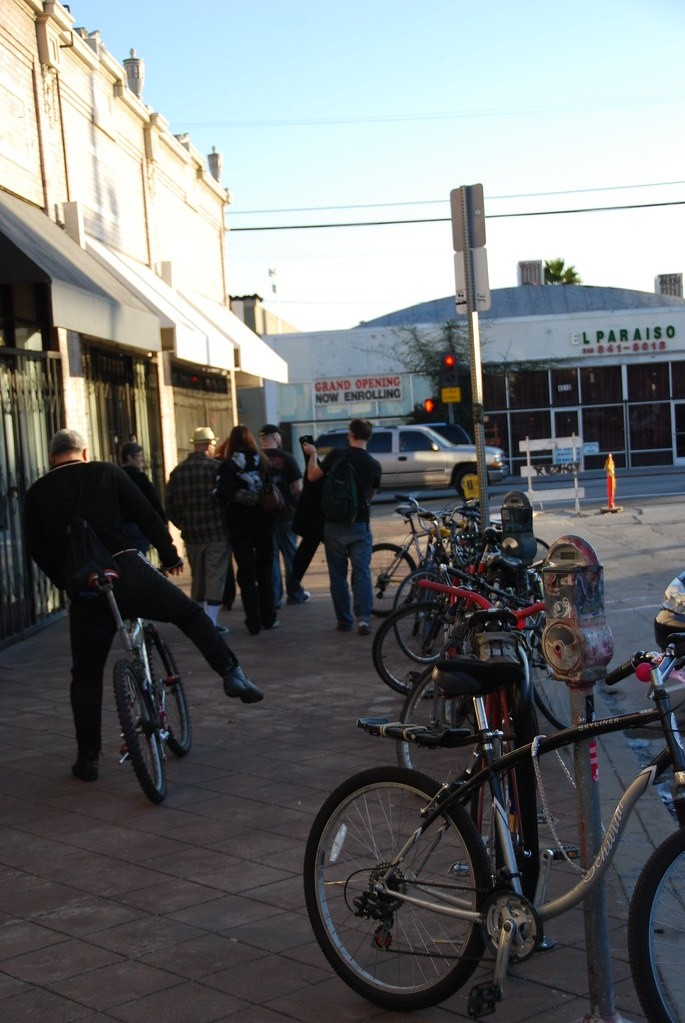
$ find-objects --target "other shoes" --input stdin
[216,625,229,634]
[302,591,311,602]
[224,664,263,703]
[358,620,372,635]
[72,750,101,782]
[336,623,352,632]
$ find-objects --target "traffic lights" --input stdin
[420,397,442,417]
[439,351,461,387]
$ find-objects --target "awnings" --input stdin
[0,191,288,386]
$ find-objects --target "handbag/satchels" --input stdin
[259,462,285,513]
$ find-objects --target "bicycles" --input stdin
[350,493,595,906]
[87,567,193,809]
[302,630,684,1022]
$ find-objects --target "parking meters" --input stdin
[536,534,625,1021]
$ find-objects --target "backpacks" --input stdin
[321,449,358,526]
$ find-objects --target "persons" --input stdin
[305,419,382,633]
[255,425,311,604]
[213,437,237,612]
[118,441,167,560]
[213,424,282,633]
[165,426,233,635]
[22,429,263,782]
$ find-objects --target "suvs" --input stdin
[311,424,510,501]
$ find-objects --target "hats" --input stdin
[190,428,219,444]
[259,424,284,437]
